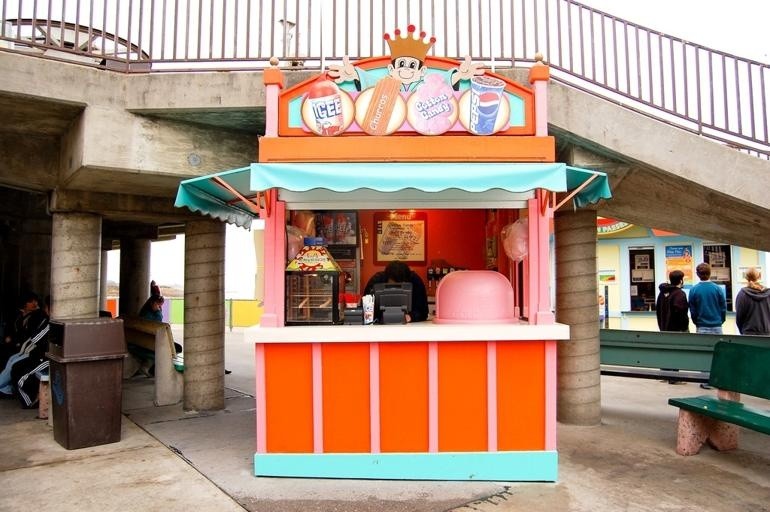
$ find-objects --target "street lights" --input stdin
[278,18,297,60]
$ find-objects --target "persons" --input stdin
[360,260,429,325]
[655,270,689,384]
[688,262,727,390]
[0,293,51,409]
[735,268,770,336]
[151,280,160,297]
[138,294,182,355]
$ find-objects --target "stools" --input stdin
[34,371,51,419]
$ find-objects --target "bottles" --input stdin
[307,81,347,137]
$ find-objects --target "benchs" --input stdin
[668,340,770,456]
[115,315,184,407]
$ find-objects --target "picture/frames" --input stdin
[373,211,428,267]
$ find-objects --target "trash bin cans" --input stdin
[46,317,128,449]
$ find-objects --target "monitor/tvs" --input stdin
[374,282,412,325]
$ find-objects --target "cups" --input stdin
[470,74,505,134]
[362,302,374,323]
[378,223,421,259]
